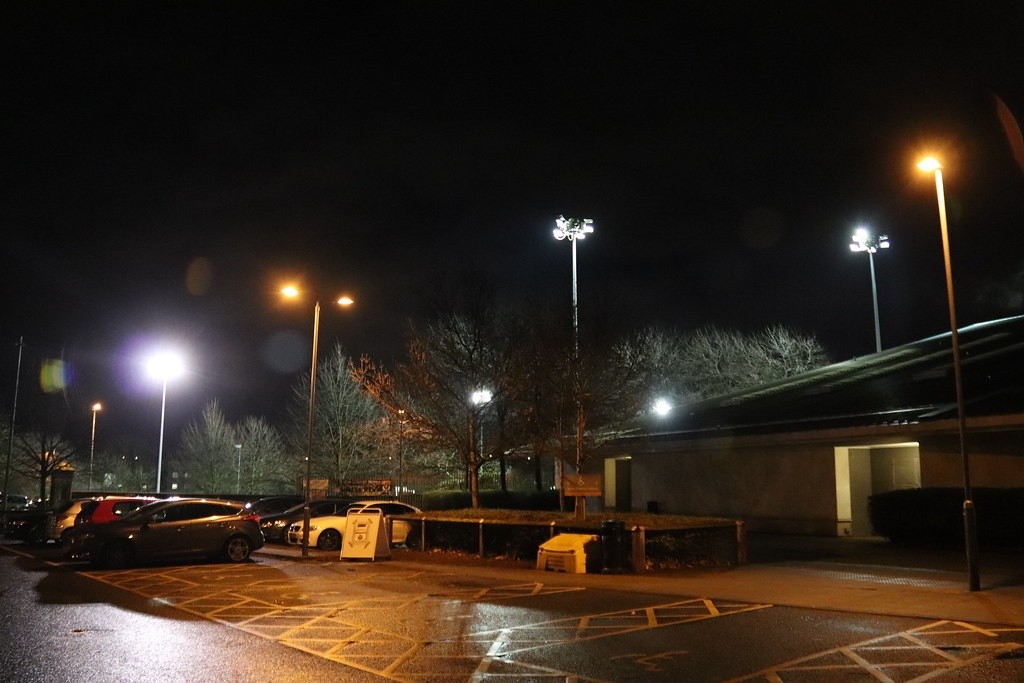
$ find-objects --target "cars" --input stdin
[45,497,100,547]
[75,496,163,530]
[0,493,58,548]
[248,496,338,545]
[287,500,428,551]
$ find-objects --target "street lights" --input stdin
[471,385,494,478]
[235,444,242,496]
[398,408,405,497]
[552,214,595,477]
[280,286,355,559]
[88,402,102,492]
[850,224,892,355]
[917,155,981,592]
[143,351,184,493]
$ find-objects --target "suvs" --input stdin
[60,497,264,569]
